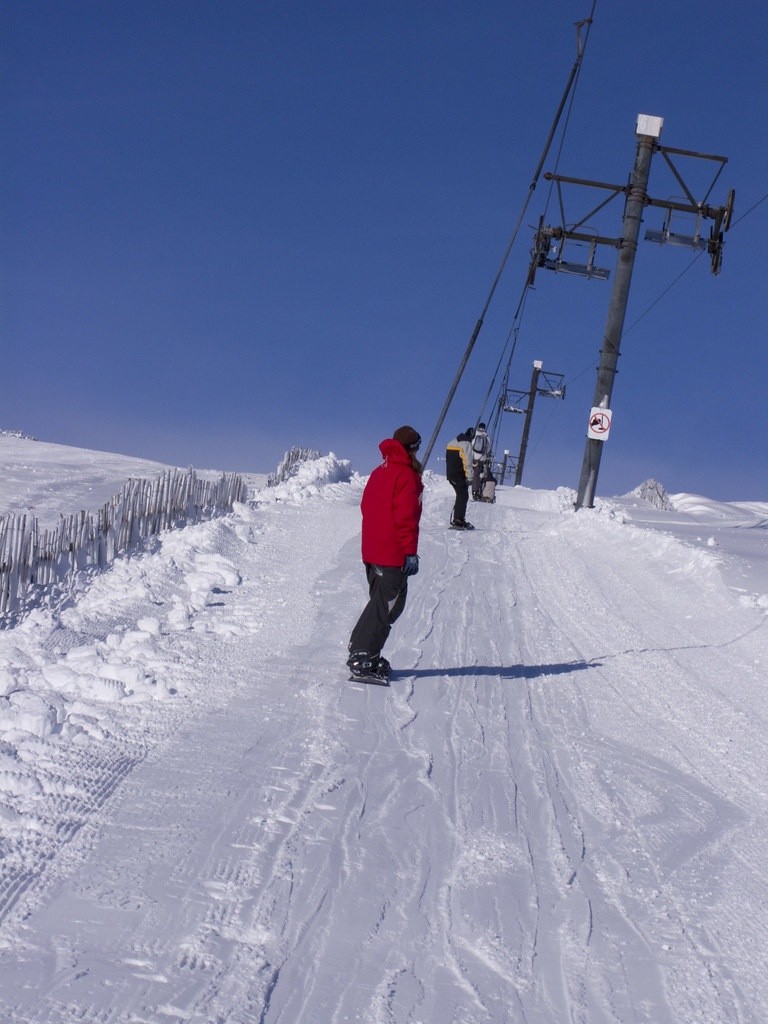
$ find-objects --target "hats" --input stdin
[393,426,421,450]
[466,427,476,434]
[479,423,486,429]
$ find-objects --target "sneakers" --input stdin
[453,519,474,530]
[346,650,393,686]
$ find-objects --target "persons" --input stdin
[446,427,476,531]
[470,423,492,501]
[346,426,425,686]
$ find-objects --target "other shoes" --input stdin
[471,490,482,501]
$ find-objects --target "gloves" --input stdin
[400,554,421,576]
[465,481,472,485]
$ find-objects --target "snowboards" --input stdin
[348,674,389,687]
[448,524,466,530]
